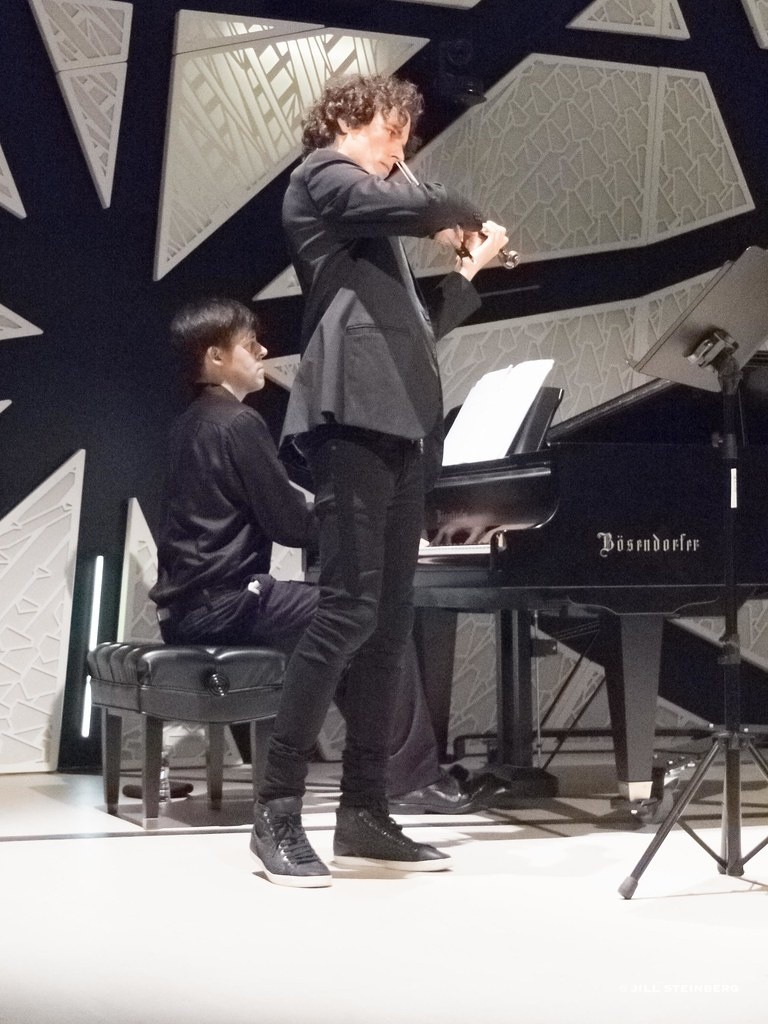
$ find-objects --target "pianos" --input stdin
[294,377,768,832]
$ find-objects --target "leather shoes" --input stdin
[387,767,496,815]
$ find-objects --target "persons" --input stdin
[244,76,509,889]
[148,299,496,817]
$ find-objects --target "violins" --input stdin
[395,163,521,273]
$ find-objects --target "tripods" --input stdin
[528,426,768,899]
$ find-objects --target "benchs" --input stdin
[86,639,287,828]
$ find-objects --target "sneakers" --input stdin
[333,804,454,872]
[249,795,333,888]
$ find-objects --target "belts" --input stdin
[156,584,226,623]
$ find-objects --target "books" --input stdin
[442,358,554,467]
